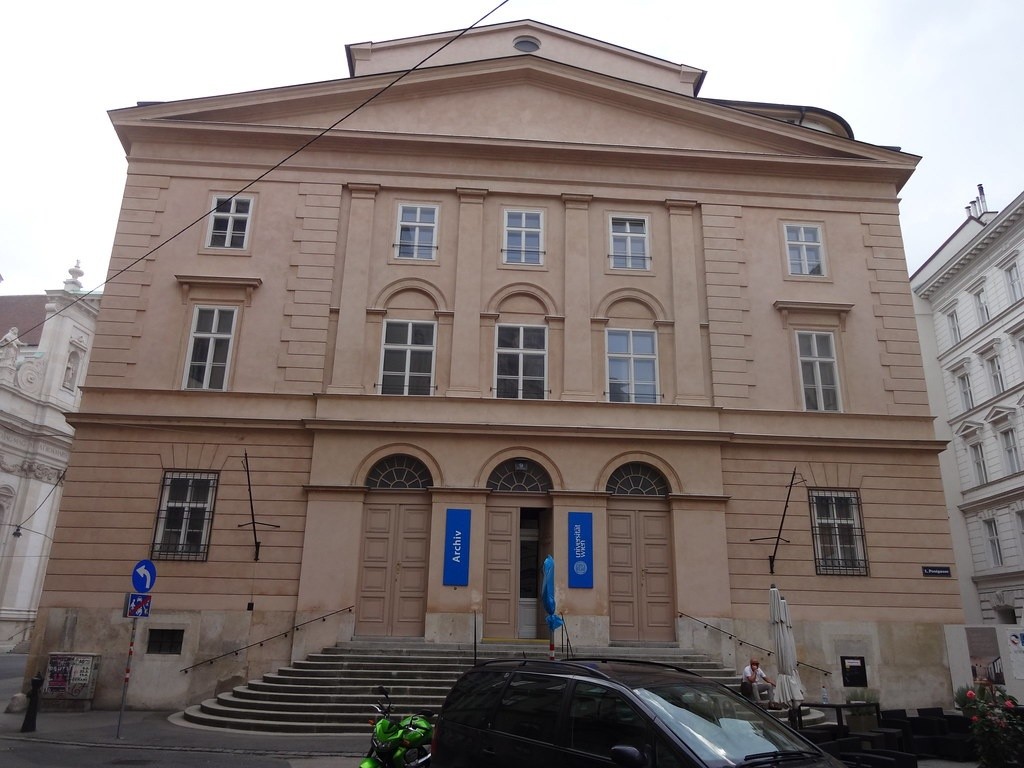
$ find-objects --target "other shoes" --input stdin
[768,704,782,710]
[758,704,766,711]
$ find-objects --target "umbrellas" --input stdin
[541,554,563,660]
[769,584,807,730]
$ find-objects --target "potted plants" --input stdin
[843,688,881,733]
[953,687,979,719]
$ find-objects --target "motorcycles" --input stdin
[359,686,434,768]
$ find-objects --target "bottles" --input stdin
[822,686,829,704]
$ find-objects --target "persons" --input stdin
[742,658,782,710]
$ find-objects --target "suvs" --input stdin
[429,658,842,768]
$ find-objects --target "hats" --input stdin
[750,658,759,665]
